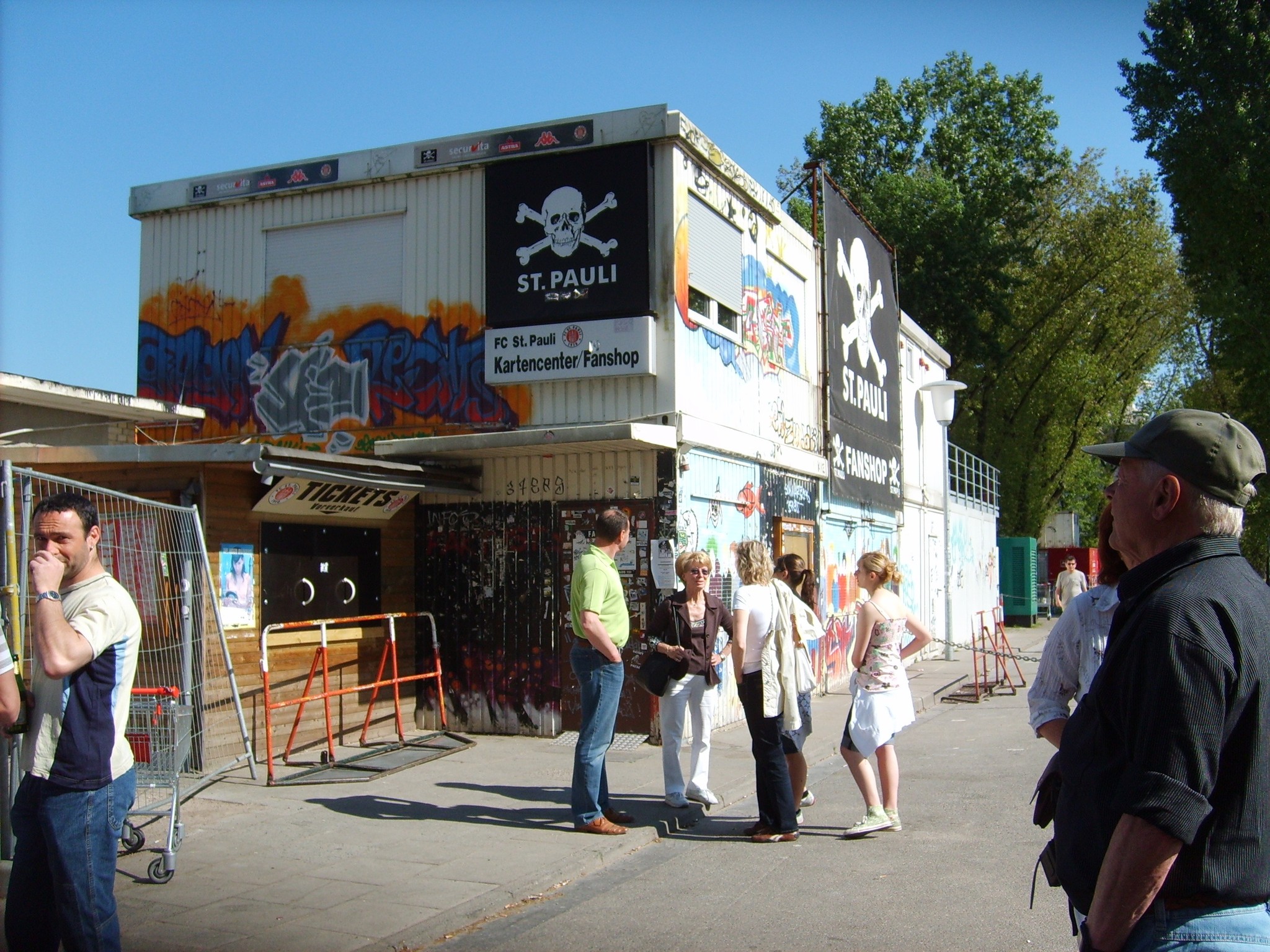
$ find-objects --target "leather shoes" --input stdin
[603,808,635,823]
[576,815,628,835]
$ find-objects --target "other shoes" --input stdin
[800,791,814,807]
[795,810,803,824]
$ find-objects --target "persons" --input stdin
[1027,503,1128,749]
[645,552,734,809]
[731,540,816,842]
[569,509,637,835]
[839,553,932,838]
[1050,408,1270,952]
[0,493,143,952]
[1055,556,1088,611]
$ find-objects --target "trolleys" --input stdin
[1037,582,1052,621]
[119,686,194,885]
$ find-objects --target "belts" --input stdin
[574,636,593,648]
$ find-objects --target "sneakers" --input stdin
[665,792,689,807]
[881,807,902,832]
[751,829,800,843]
[843,804,891,838]
[685,788,718,805]
[744,820,767,836]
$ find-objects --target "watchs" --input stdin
[35,590,62,603]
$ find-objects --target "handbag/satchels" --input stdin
[634,651,671,697]
[789,606,817,694]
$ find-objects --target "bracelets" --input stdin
[718,652,726,661]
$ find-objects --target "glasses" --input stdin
[686,569,709,575]
[1066,562,1076,565]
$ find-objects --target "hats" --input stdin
[1080,409,1267,509]
